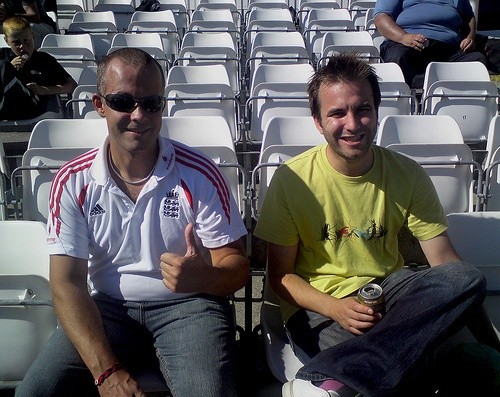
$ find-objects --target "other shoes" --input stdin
[282,377,341,397]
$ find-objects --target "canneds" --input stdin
[357,284,385,333]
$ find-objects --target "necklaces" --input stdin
[108,149,157,184]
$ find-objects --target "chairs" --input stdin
[0,0,500,397]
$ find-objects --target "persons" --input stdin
[0,0,80,191]
[12,47,251,397]
[251,49,487,397]
[374,0,487,103]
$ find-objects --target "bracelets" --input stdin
[95,362,122,386]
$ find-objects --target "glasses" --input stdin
[101,92,167,113]
